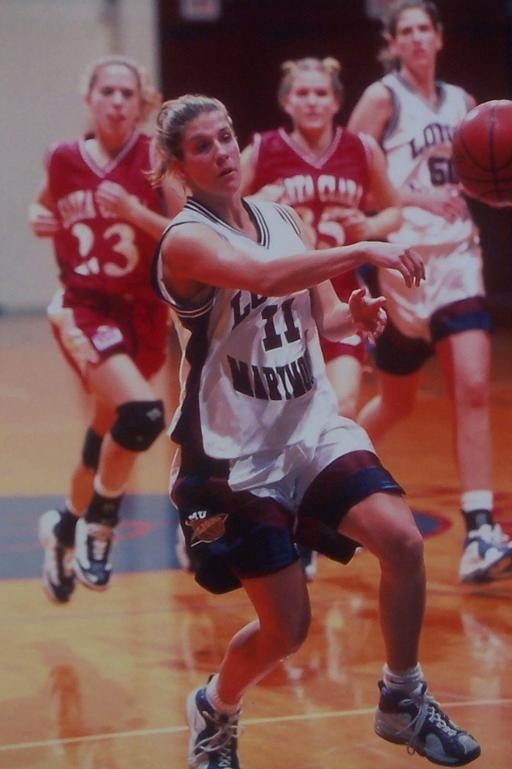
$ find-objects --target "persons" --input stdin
[28,59,188,605]
[346,0,512,584]
[142,91,482,769]
[236,55,404,583]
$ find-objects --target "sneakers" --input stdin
[37,510,82,605]
[456,522,510,580]
[370,679,482,767]
[76,517,118,588]
[183,672,245,768]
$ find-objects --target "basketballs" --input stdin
[453,99,510,209]
